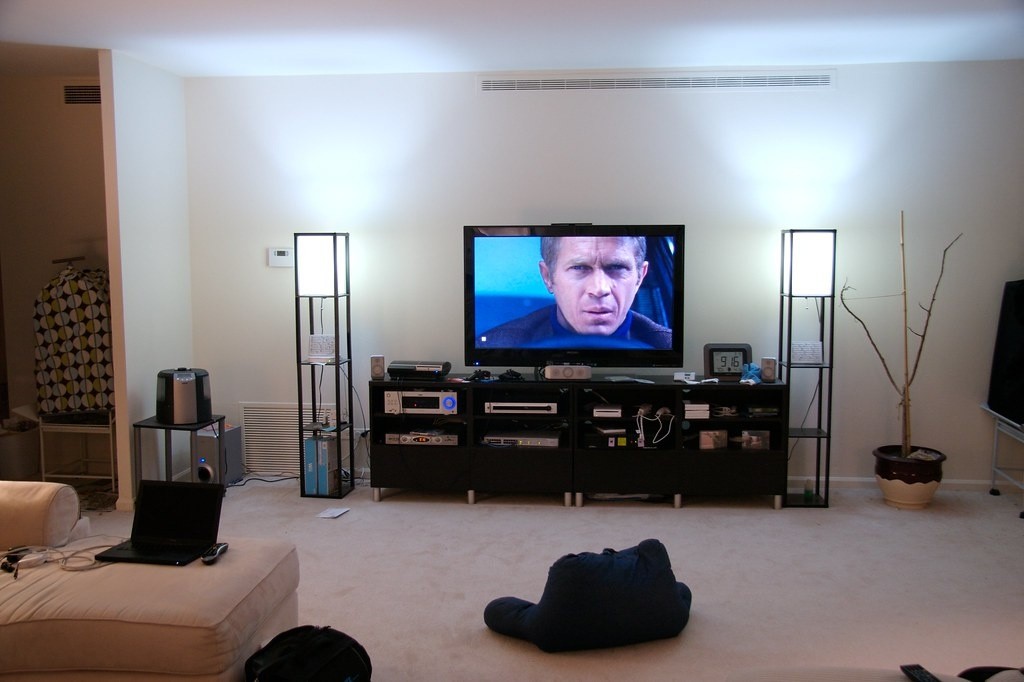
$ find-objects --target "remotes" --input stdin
[200,543,229,566]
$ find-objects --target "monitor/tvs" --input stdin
[462,224,685,368]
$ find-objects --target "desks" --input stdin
[134,415,226,498]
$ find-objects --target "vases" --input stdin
[871,444,946,510]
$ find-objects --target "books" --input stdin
[317,508,349,517]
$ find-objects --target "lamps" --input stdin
[293,233,356,499]
[778,229,837,508]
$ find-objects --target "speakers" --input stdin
[761,357,776,382]
[196,425,243,488]
[544,365,592,380]
[371,355,384,380]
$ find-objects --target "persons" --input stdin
[475,237,673,349]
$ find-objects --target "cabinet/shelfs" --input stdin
[12,404,117,492]
[369,372,787,510]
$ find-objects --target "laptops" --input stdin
[94,480,226,566]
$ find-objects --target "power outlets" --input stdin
[332,428,370,440]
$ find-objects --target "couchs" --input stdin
[0,480,299,682]
